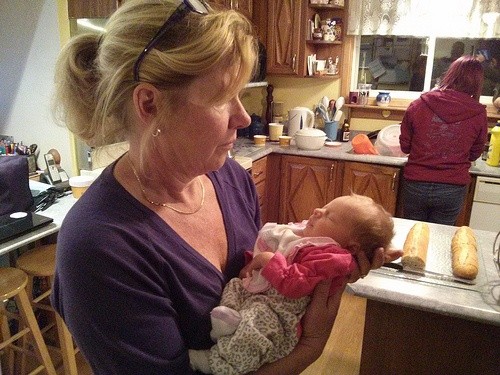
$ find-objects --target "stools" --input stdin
[0,244,81,375]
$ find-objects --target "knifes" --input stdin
[383,263,476,285]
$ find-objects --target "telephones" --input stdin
[42,154,70,186]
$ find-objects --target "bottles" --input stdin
[376,92,391,106]
[486,121,500,166]
[340,119,349,143]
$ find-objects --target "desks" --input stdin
[345,216,500,375]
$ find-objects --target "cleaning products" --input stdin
[485,120,500,167]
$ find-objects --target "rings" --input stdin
[360,275,366,278]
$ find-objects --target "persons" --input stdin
[49,0,404,375]
[399,55,488,226]
[409,43,500,96]
[188,187,396,375]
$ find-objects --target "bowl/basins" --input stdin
[294,128,325,152]
[375,124,412,158]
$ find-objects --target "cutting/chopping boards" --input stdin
[370,218,489,294]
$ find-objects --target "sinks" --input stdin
[346,140,407,157]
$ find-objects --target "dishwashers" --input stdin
[469,176,500,234]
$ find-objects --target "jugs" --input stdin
[351,135,379,155]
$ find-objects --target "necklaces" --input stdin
[127,153,205,215]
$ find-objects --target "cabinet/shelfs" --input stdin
[68,0,349,77]
[247,152,477,226]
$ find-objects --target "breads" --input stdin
[451,225,479,279]
[401,222,430,269]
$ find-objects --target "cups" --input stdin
[358,84,371,106]
[253,135,266,145]
[326,121,338,142]
[269,122,284,144]
[349,91,360,105]
[70,176,91,200]
[280,137,291,148]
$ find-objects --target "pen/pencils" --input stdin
[0,139,31,155]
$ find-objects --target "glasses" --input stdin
[134,0,216,83]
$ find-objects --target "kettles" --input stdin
[287,107,315,140]
